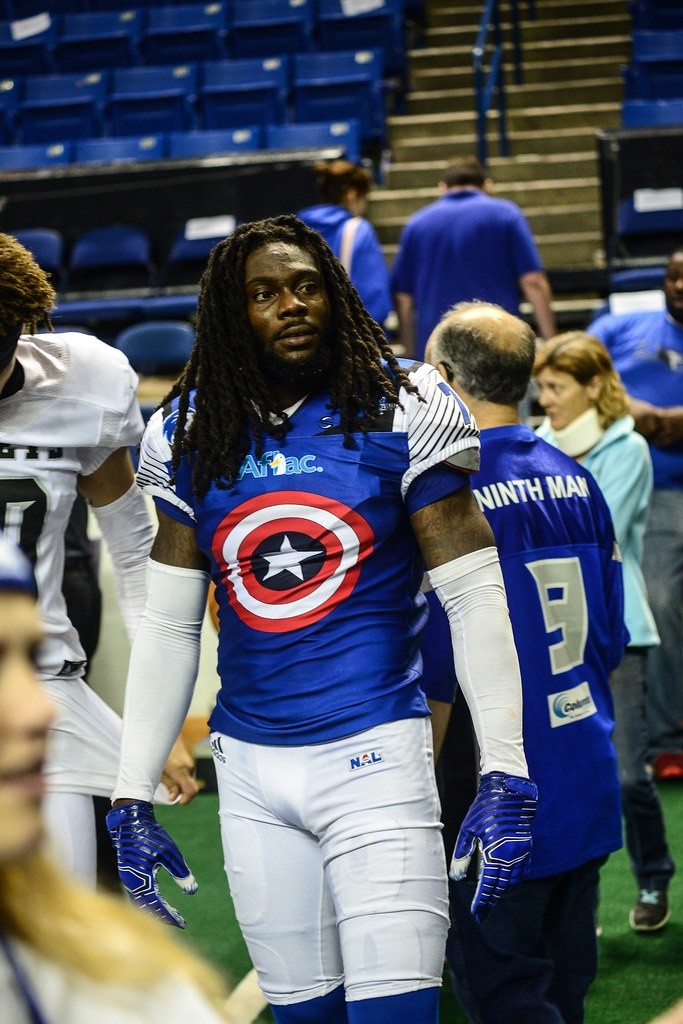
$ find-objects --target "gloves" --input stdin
[103,800,200,929]
[449,772,538,924]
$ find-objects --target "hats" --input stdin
[0,530,38,596]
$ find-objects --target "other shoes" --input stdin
[655,752,682,780]
[194,755,220,794]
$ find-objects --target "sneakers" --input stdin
[629,885,670,931]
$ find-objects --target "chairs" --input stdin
[0,0,683,489]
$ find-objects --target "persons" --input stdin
[0,157,683,1024]
